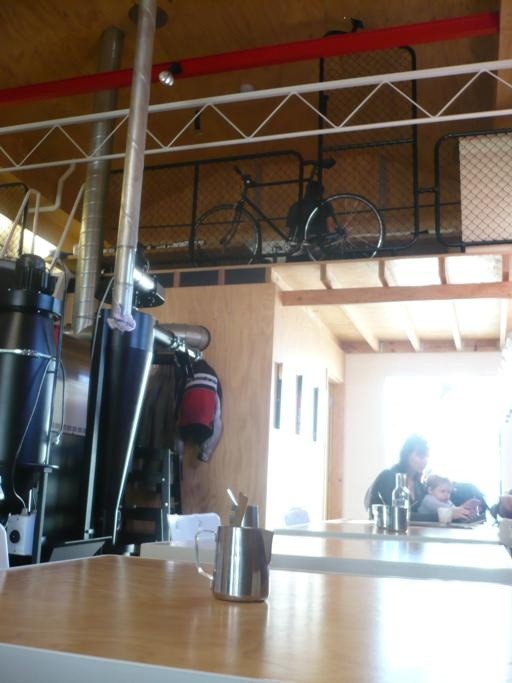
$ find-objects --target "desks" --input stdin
[0,517,512,683]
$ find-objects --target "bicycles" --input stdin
[188,158,385,267]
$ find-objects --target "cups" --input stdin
[386,506,409,534]
[371,505,394,529]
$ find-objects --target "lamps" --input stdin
[158,61,181,87]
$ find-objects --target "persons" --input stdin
[367,432,482,523]
[416,475,460,513]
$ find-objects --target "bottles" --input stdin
[192,523,276,601]
[391,472,410,533]
